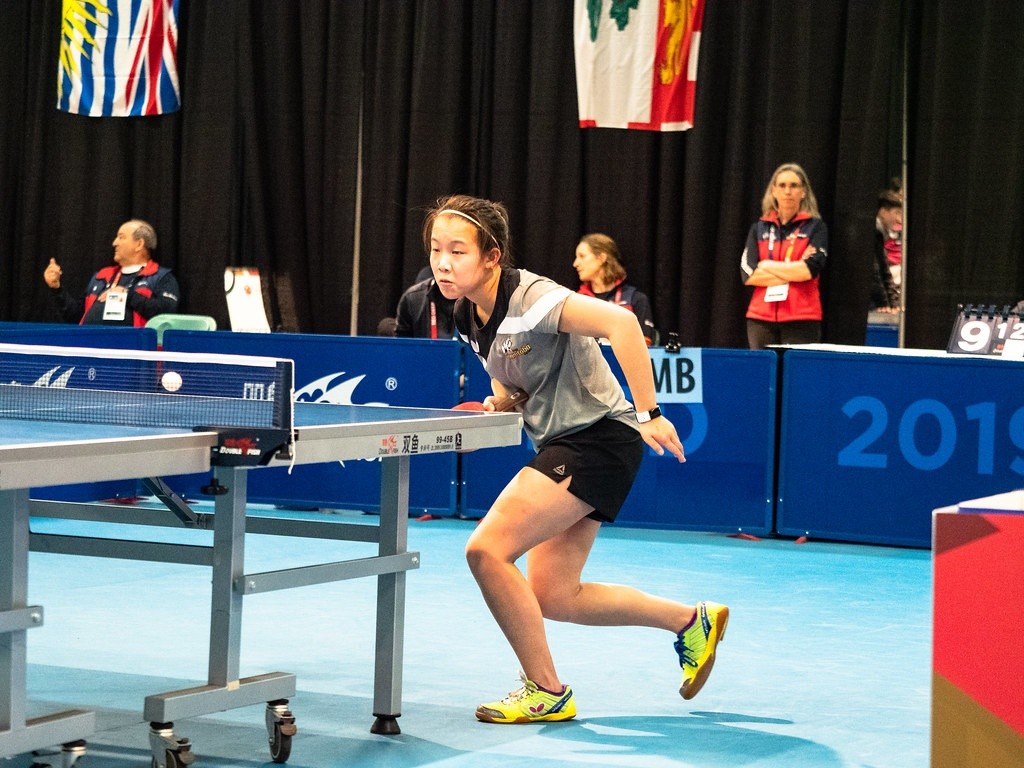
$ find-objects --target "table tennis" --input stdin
[162,370,183,393]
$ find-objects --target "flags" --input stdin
[56,0,182,118]
[573,0,703,133]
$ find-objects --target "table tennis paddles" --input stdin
[448,387,530,413]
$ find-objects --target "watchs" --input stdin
[635,405,662,424]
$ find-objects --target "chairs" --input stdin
[145,313,217,346]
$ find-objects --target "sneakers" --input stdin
[673,600,730,700]
[476,671,576,722]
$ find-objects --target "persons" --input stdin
[378,266,434,337]
[841,191,903,345]
[572,233,656,348]
[882,176,903,265]
[740,164,831,351]
[45,219,182,329]
[423,195,729,724]
[395,276,461,341]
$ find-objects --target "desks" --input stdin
[0,343,524,767]
[930,489,1024,768]
[765,343,1024,362]
